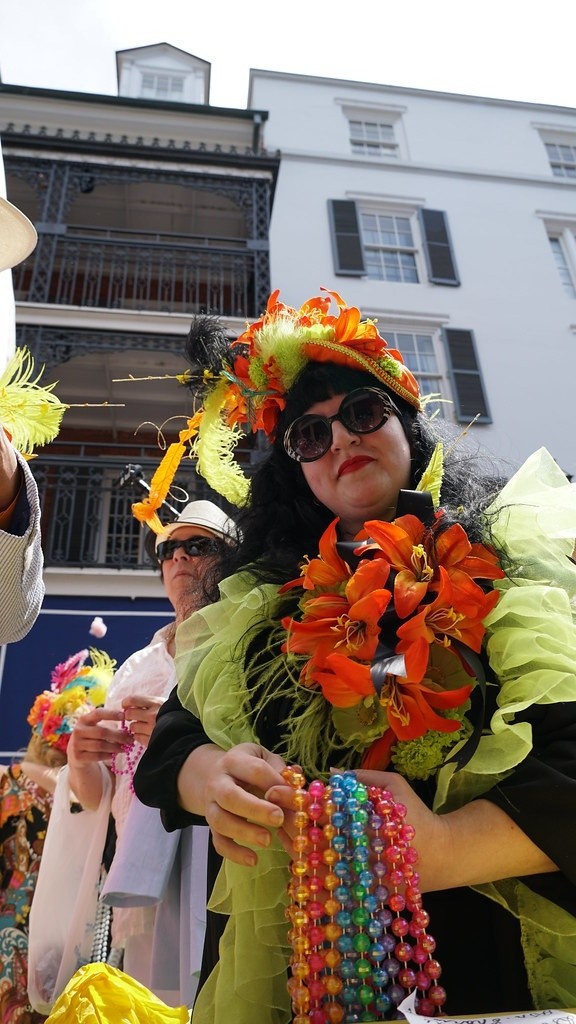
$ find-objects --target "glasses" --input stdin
[284,386,402,462]
[157,536,221,560]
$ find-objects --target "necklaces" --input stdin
[282,767,445,1024]
[111,706,144,797]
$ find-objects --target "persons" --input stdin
[0,353,66,648]
[134,288,576,1024]
[0,649,116,1024]
[27,500,242,1017]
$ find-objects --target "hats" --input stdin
[154,500,243,564]
[112,284,422,508]
[0,197,38,273]
